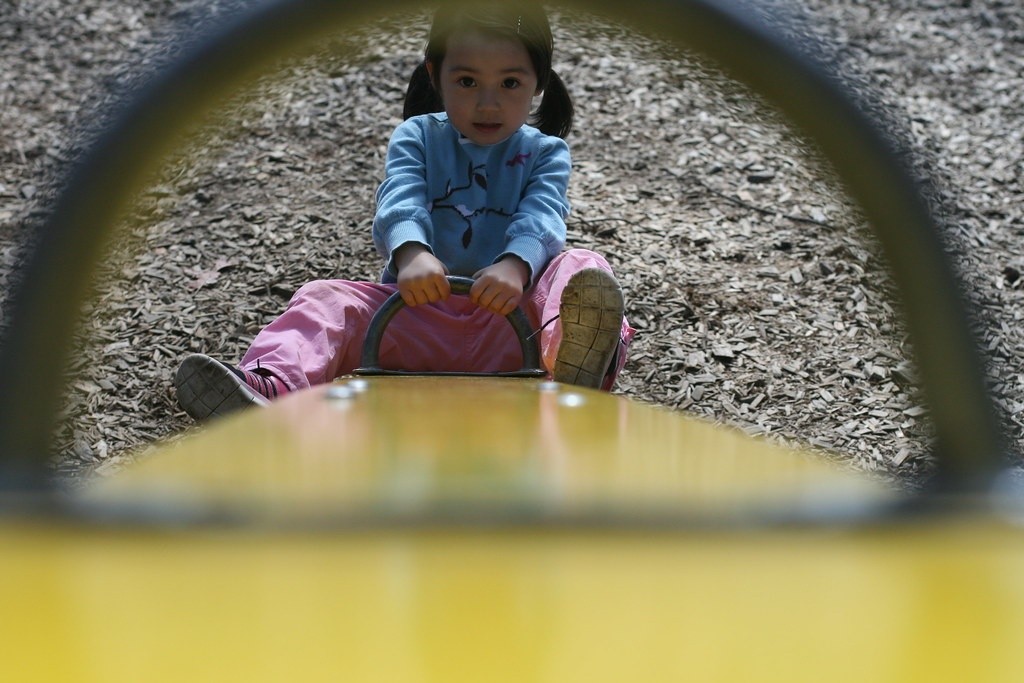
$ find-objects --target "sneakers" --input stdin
[175,353,291,425]
[526,268,624,390]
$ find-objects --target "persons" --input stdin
[174,0,637,424]
